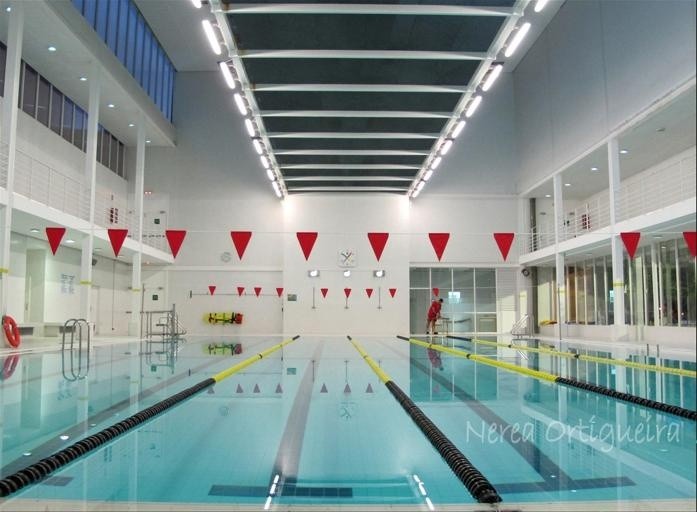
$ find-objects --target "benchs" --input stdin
[10,322,96,337]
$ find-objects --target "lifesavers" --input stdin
[2,351,18,379]
[2,316,20,348]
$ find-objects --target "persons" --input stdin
[425,298,444,335]
[426,337,443,372]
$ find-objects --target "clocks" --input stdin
[338,248,358,267]
